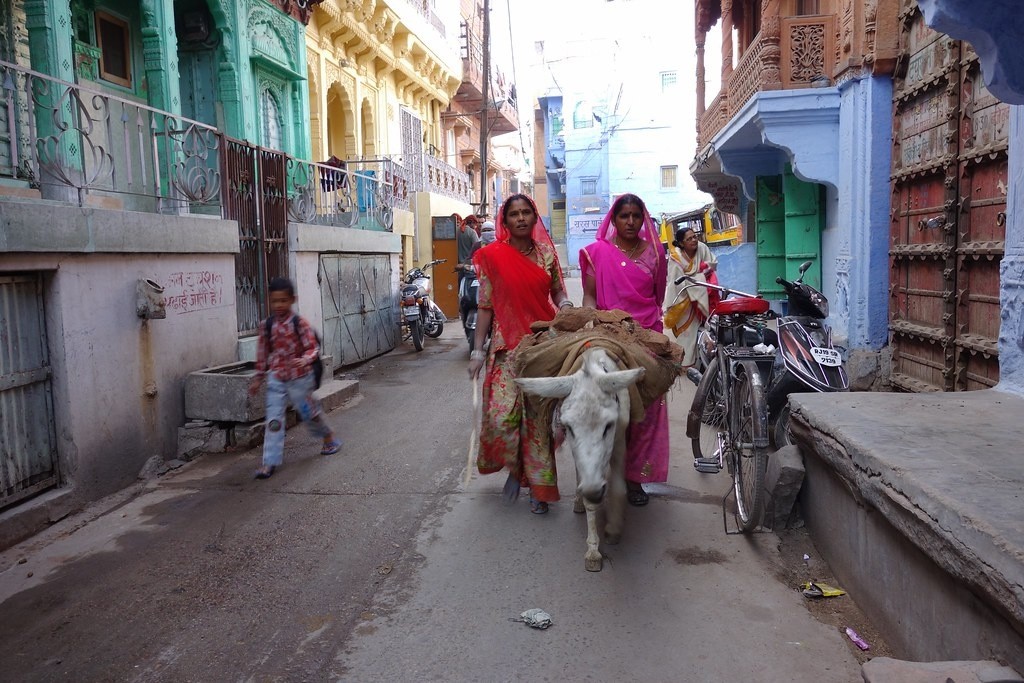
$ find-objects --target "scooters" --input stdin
[451,240,495,354]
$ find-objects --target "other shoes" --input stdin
[627,486,650,506]
[501,475,520,505]
[529,491,550,515]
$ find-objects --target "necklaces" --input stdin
[614,238,638,260]
[576,193,669,513]
[508,238,535,257]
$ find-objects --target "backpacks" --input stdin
[267,315,325,392]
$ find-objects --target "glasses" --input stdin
[683,235,699,242]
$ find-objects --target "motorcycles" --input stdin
[686,262,851,451]
[399,259,451,350]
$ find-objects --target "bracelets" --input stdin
[470,350,487,361]
[558,300,574,310]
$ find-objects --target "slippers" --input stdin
[321,440,342,455]
[255,464,277,478]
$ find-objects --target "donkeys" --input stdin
[513,311,659,573]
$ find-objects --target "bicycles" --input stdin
[672,274,775,536]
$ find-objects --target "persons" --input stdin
[662,228,720,376]
[452,212,496,313]
[466,195,575,514]
[247,278,340,478]
